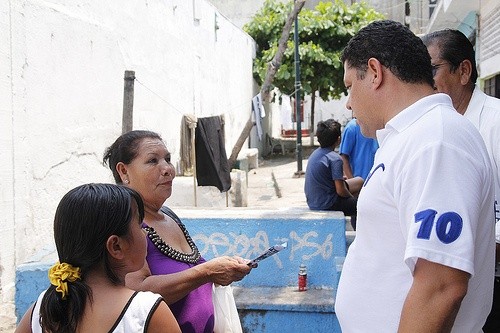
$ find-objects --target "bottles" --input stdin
[298,264,307,291]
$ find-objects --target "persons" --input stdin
[419,29,500,333]
[14,184,182,333]
[335,22,495,333]
[109,130,258,333]
[339,117,379,180]
[304,119,365,231]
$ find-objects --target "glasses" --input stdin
[431,63,459,76]
